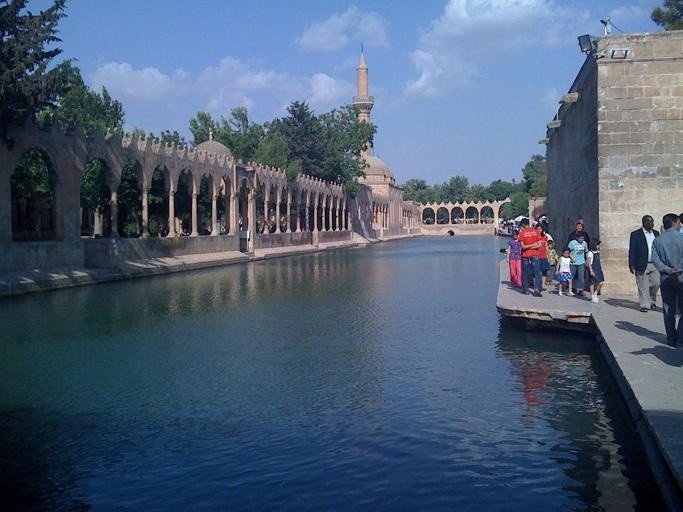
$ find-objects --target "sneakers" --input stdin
[558,292,585,296]
[591,298,599,303]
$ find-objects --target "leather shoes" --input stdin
[521,290,543,297]
[641,304,656,312]
[669,343,683,347]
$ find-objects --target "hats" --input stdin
[576,215,583,219]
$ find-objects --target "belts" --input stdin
[648,262,653,263]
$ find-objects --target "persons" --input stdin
[651,213,683,346]
[501,214,605,303]
[629,215,660,313]
